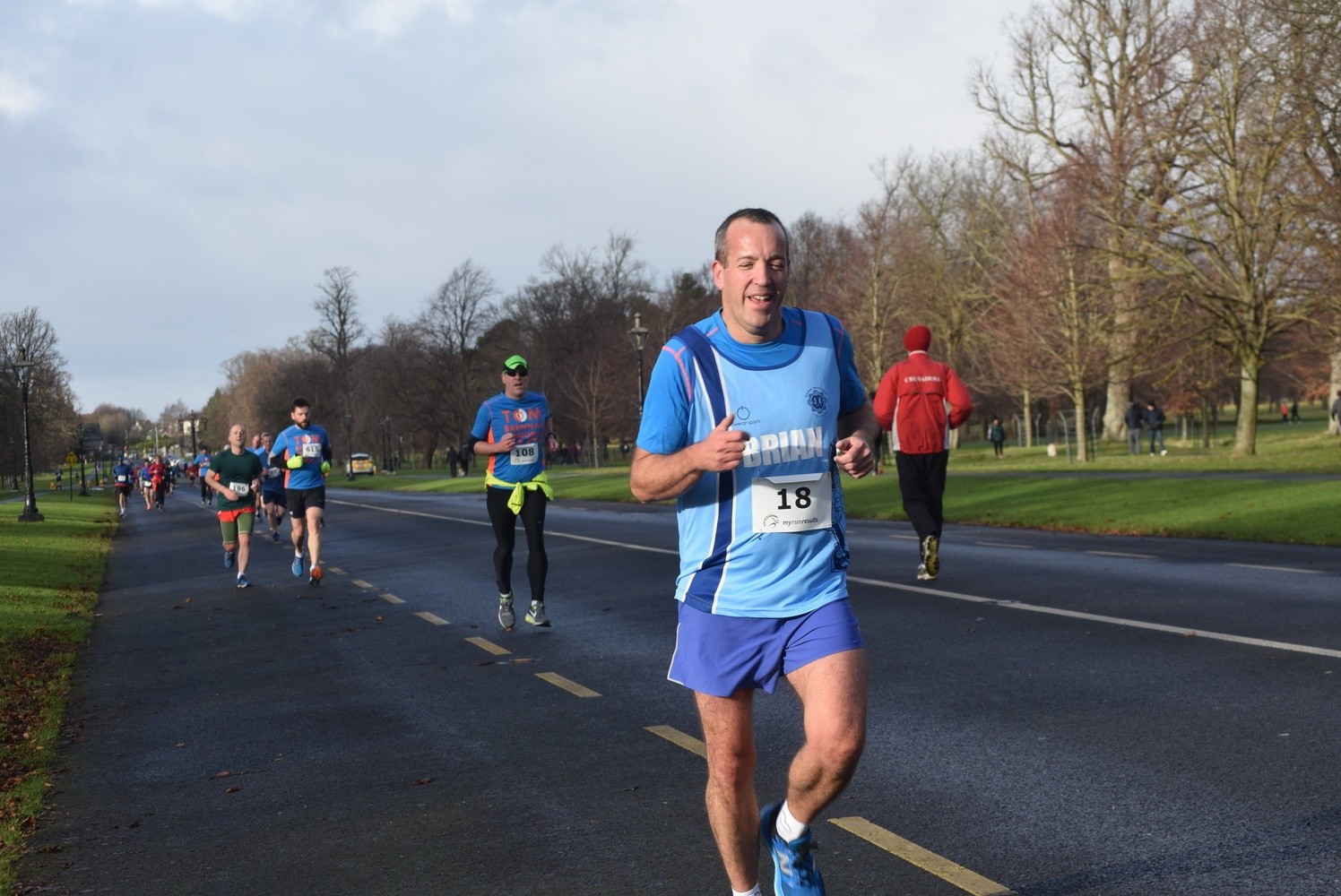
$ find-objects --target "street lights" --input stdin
[628,313,648,417]
[74,422,89,495]
[381,414,396,475]
[11,344,44,522]
[189,408,199,461]
[344,408,355,481]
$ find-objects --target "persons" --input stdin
[467,355,558,630]
[204,424,267,588]
[253,432,287,542]
[628,206,884,895]
[380,429,636,482]
[871,324,975,583]
[265,397,332,586]
[868,386,1339,476]
[52,432,266,522]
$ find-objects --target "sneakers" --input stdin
[759,798,827,896]
[292,546,305,577]
[525,601,550,626]
[237,574,251,588]
[309,566,323,587]
[917,563,937,580]
[498,588,516,630]
[922,535,940,576]
[224,549,236,568]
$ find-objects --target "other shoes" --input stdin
[150,499,154,504]
[254,513,263,522]
[208,501,212,506]
[276,516,282,525]
[202,499,205,505]
[1160,450,1168,456]
[263,509,267,518]
[1150,453,1154,456]
[120,514,127,517]
[273,532,280,541]
[147,505,151,510]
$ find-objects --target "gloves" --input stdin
[320,461,331,473]
[286,454,303,469]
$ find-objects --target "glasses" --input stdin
[502,369,529,377]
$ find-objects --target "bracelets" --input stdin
[256,478,263,487]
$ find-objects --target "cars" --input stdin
[345,453,376,477]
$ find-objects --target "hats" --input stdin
[904,325,931,352]
[201,445,207,450]
[503,355,529,371]
[1148,401,1155,406]
[118,456,123,460]
